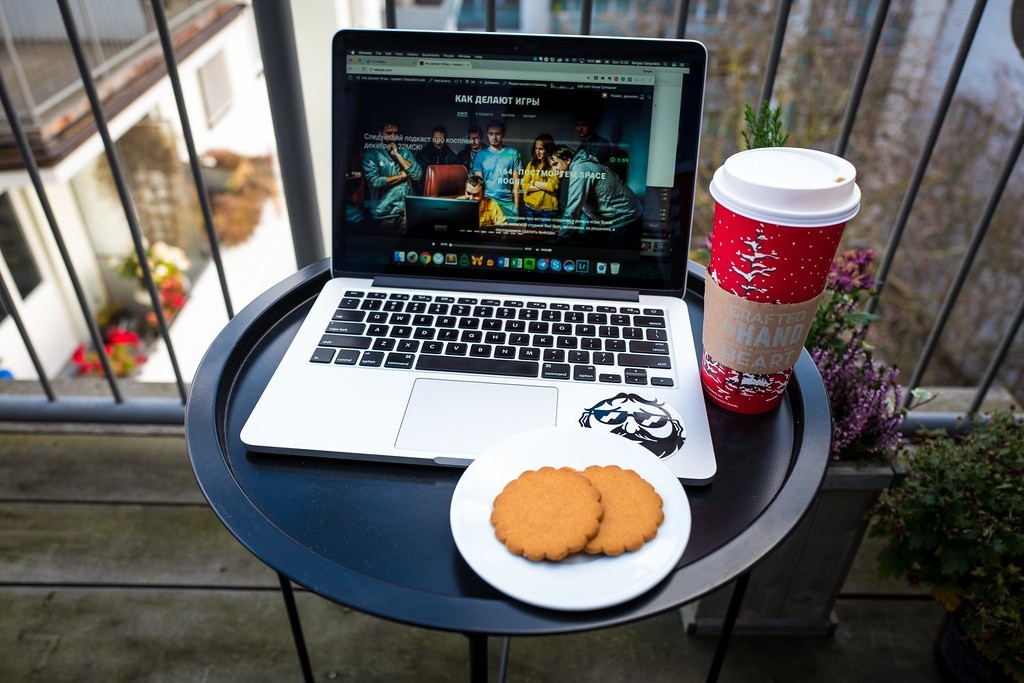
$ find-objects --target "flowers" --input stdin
[691,102,943,465]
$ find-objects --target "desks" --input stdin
[185,258,832,683]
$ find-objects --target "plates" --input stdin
[450,427,691,611]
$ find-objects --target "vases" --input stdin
[681,464,894,637]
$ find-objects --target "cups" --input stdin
[700,147,861,414]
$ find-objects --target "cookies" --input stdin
[576,465,665,556]
[490,466,604,561]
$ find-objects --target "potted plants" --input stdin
[866,403,1023,682]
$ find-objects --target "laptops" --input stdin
[406,195,479,232]
[240,30,719,485]
